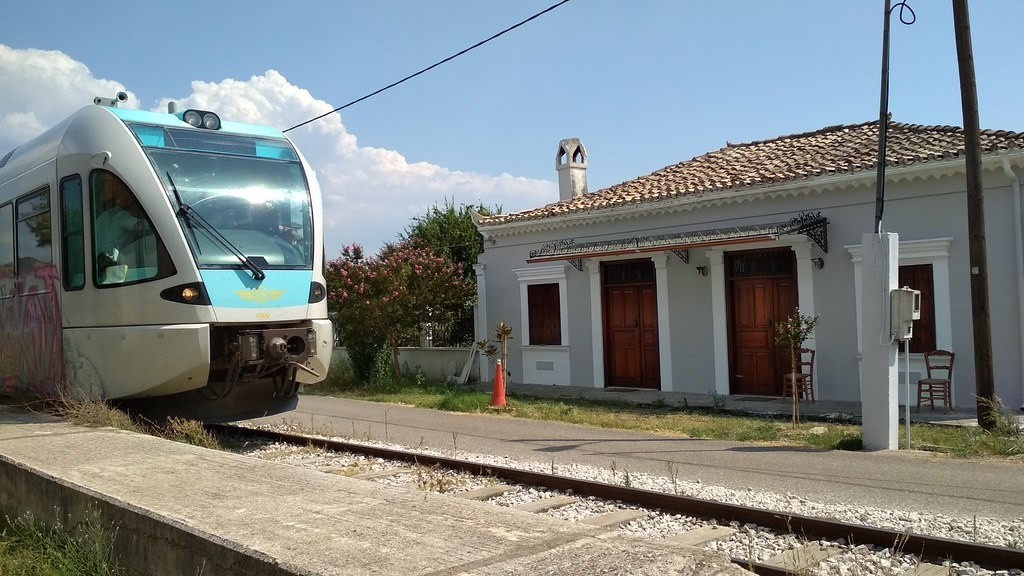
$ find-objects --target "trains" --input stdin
[0,91,332,424]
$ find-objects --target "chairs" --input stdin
[916,350,955,413]
[783,347,816,403]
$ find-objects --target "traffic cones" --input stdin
[490,359,508,409]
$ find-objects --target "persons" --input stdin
[267,209,298,243]
[96,192,144,278]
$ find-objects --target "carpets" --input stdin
[605,388,640,394]
[734,396,777,402]
